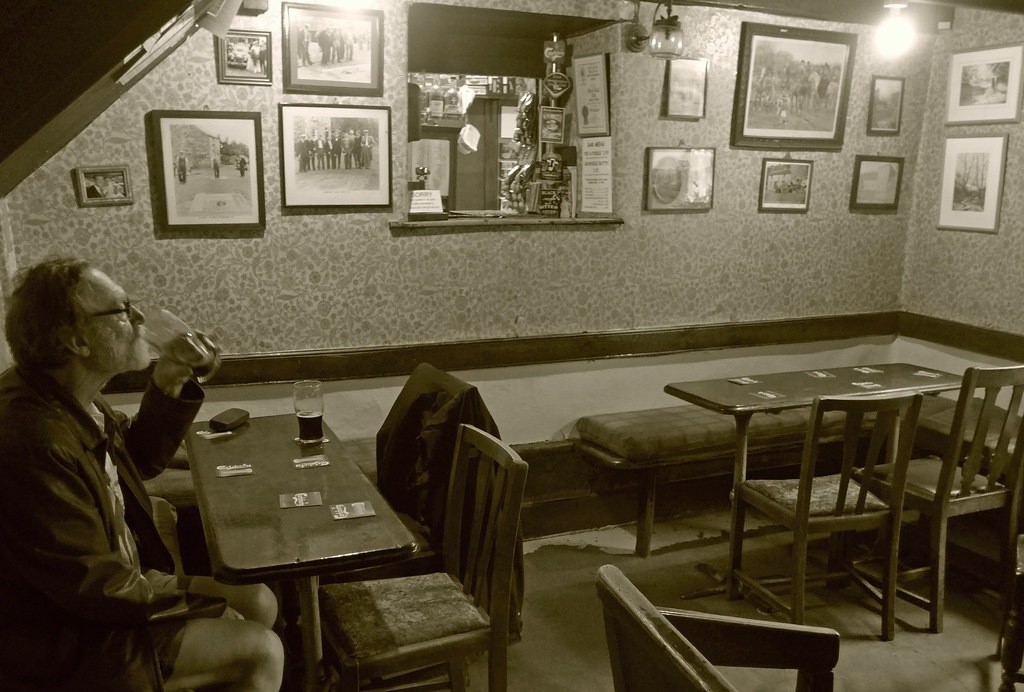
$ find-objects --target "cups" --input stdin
[292,380,325,445]
[139,308,228,383]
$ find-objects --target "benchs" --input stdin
[144,440,377,511]
[576,396,1024,602]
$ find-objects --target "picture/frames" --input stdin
[943,41,1024,125]
[729,22,858,151]
[281,2,385,96]
[865,75,904,135]
[667,57,708,118]
[848,155,905,209]
[75,30,273,228]
[646,147,716,212]
[758,158,814,213]
[936,133,1009,234]
[278,102,392,208]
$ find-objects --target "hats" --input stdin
[305,23,310,28]
[363,130,368,133]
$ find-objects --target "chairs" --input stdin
[597,563,841,692]
[310,364,530,692]
[855,365,1024,633]
[729,393,923,642]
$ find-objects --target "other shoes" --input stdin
[310,62,315,65]
[338,60,342,63]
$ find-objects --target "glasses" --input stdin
[89,298,130,319]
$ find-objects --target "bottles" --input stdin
[416,72,461,120]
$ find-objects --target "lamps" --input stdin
[627,0,684,60]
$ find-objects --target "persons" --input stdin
[773,175,808,195]
[758,56,832,110]
[299,22,354,65]
[246,39,268,74]
[86,176,125,199]
[0,260,284,692]
[174,148,247,184]
[295,127,374,173]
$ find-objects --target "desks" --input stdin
[664,364,963,602]
[182,413,418,692]
